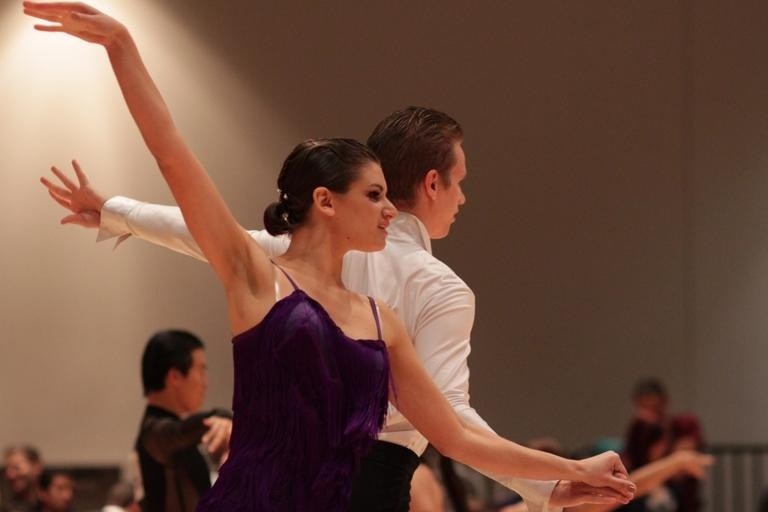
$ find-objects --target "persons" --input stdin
[102,482,135,512]
[409,443,529,511]
[40,108,634,512]
[134,328,212,512]
[207,408,232,468]
[623,377,709,511]
[23,1,637,512]
[525,438,714,511]
[0,445,52,512]
[36,470,74,511]
[184,411,221,489]
